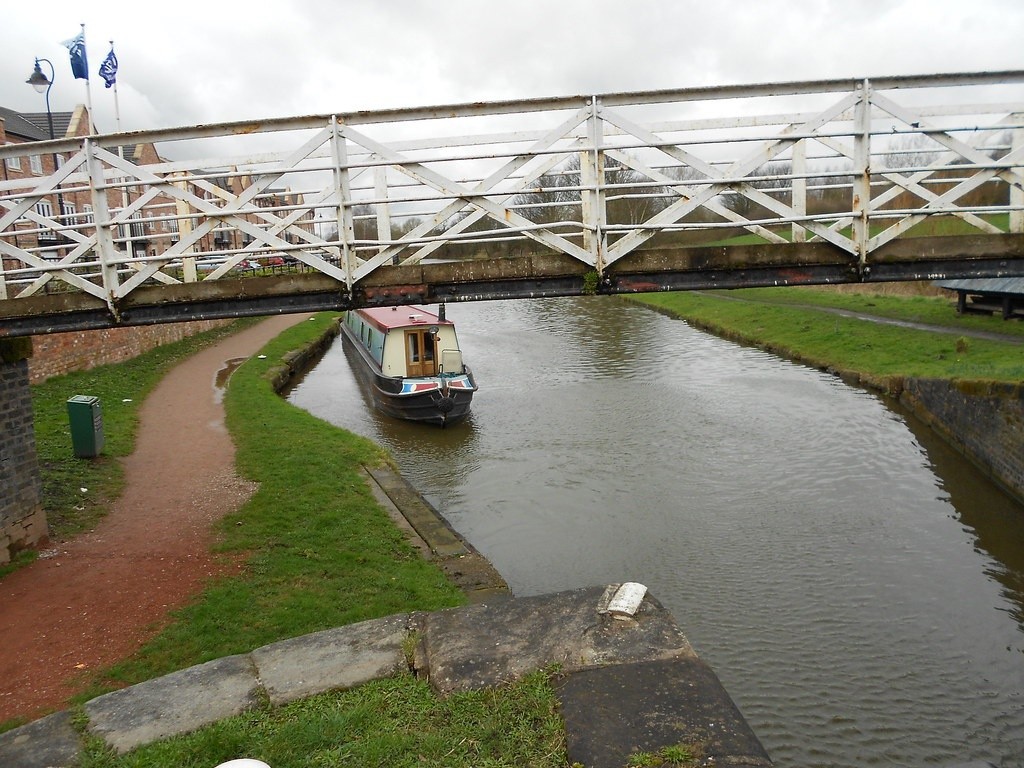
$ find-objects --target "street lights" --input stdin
[226,184,237,249]
[26,56,70,257]
[318,212,323,238]
[119,177,135,258]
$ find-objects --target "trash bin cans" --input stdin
[67,395,104,459]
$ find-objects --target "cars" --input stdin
[197,247,339,271]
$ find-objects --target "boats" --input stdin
[340,304,479,431]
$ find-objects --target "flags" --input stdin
[59,31,89,80]
[98,50,119,89]
